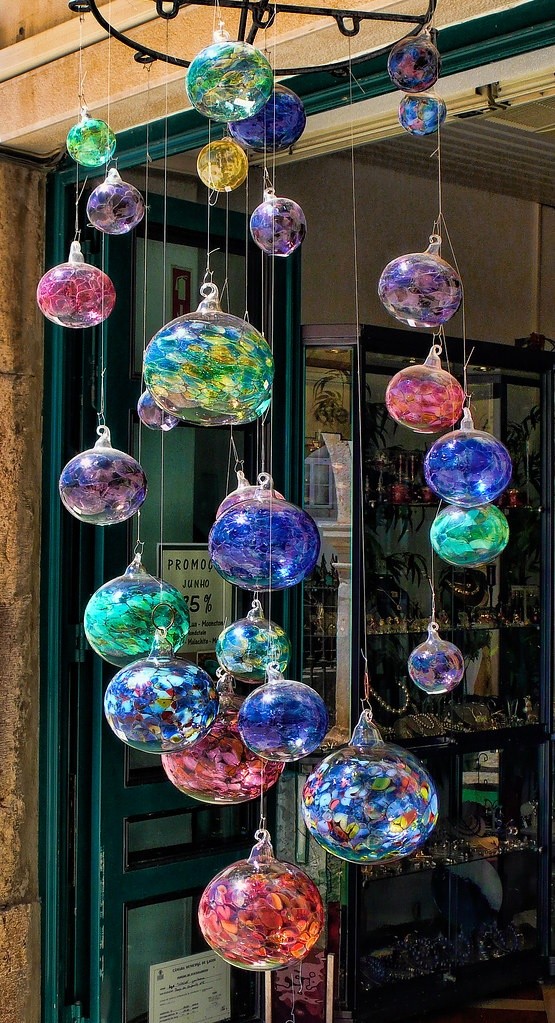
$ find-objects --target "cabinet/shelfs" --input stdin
[262,322,553,1022]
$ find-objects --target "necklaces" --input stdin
[367,681,410,714]
[374,583,402,611]
[444,575,480,595]
[463,703,487,722]
[412,713,444,737]
[446,816,491,839]
